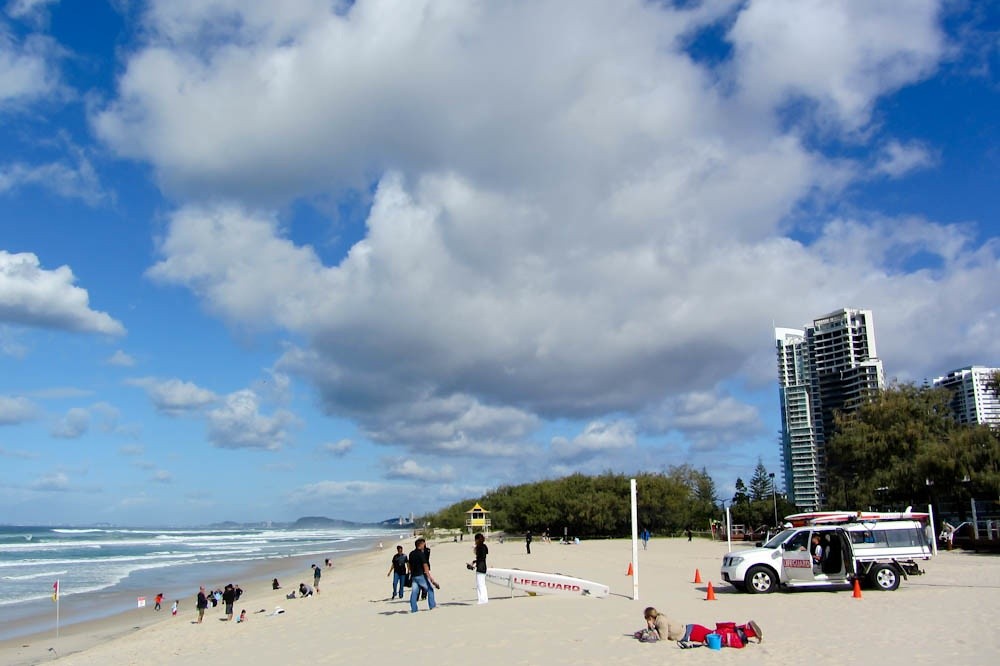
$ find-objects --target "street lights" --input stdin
[769,472,778,529]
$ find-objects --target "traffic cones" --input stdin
[695,569,701,583]
[628,562,633,576]
[705,582,718,600]
[851,580,862,598]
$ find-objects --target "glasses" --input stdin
[645,615,652,619]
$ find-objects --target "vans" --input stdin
[838,524,931,560]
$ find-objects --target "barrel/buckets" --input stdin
[706,634,721,650]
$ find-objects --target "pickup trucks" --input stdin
[720,522,924,594]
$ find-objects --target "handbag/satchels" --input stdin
[704,628,743,649]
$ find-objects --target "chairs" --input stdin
[813,534,831,573]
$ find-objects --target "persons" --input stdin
[409,538,436,612]
[472,534,489,604]
[526,530,532,554]
[575,537,579,544]
[799,534,822,564]
[155,594,162,610]
[197,583,246,623]
[172,600,179,616]
[688,529,692,541]
[325,558,331,567]
[925,519,933,545]
[312,565,321,593]
[499,536,504,544]
[288,591,295,599]
[780,522,785,531]
[388,545,409,599]
[300,584,314,598]
[273,579,279,589]
[942,520,955,544]
[543,528,550,542]
[645,607,763,648]
[641,528,649,550]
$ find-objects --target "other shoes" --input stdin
[735,627,749,644]
[749,620,762,640]
